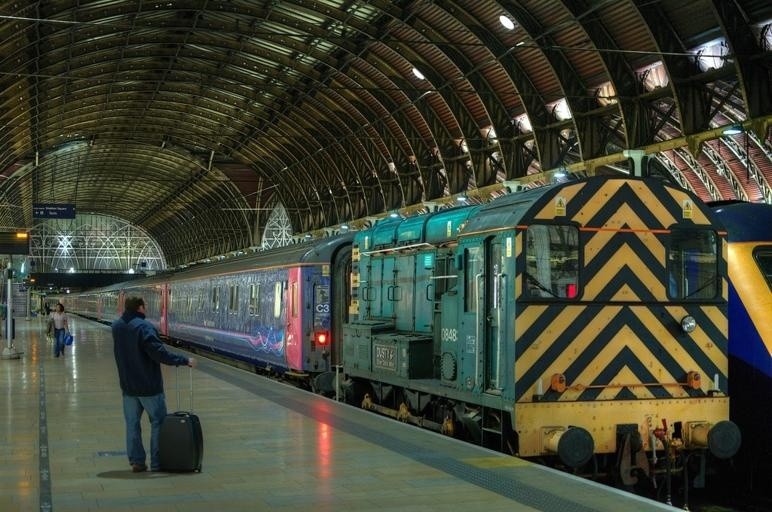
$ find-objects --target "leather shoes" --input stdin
[130,463,148,473]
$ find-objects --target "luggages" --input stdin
[158,362,204,475]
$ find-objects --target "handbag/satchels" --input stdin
[64,335,73,346]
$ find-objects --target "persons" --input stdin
[109,295,199,474]
[49,303,71,358]
[42,298,50,316]
